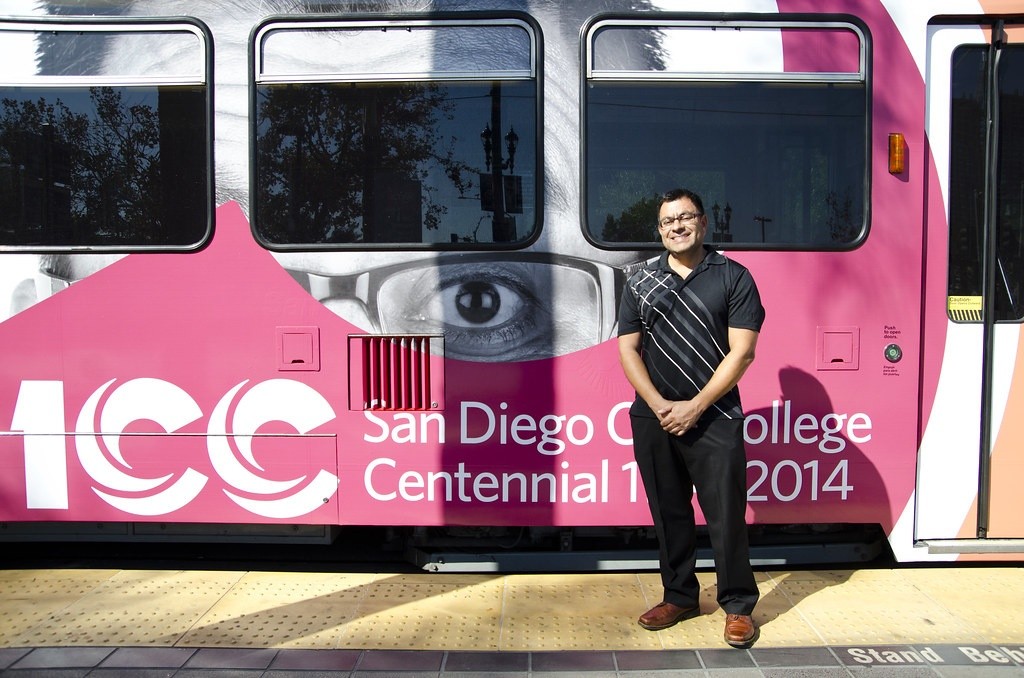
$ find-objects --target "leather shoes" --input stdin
[724,613,756,646]
[638,601,700,630]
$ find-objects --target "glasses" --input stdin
[659,212,702,230]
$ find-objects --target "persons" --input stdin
[34,0,669,362]
[617,188,766,645]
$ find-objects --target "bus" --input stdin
[0,0,1024,573]
